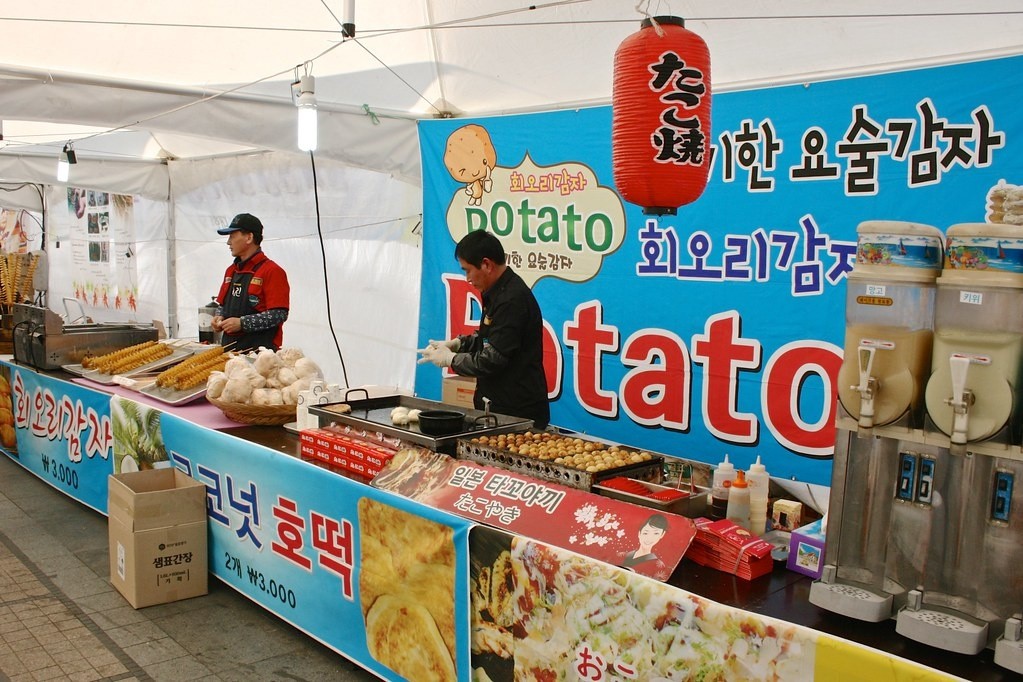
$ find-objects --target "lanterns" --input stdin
[611,16,712,218]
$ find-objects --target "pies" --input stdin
[357,497,457,681]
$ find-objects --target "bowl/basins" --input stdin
[419,410,466,436]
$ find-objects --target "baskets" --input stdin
[206,393,296,424]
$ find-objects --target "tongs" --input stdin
[678,464,694,493]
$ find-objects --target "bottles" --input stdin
[712,454,737,520]
[747,455,769,536]
[727,470,750,531]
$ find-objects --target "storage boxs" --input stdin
[108,466,210,609]
[787,517,829,577]
[298,427,393,480]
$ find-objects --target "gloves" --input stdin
[422,338,461,358]
[417,339,456,368]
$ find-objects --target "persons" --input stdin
[210,213,290,353]
[415,228,550,432]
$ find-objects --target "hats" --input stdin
[217,213,263,235]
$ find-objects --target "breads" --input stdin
[207,346,320,406]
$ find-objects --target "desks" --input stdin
[0,352,1023,682]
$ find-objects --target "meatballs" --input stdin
[470,432,652,472]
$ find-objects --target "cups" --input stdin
[296,381,340,430]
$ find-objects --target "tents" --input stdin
[1,63,445,401]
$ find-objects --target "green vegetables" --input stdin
[110,395,167,475]
